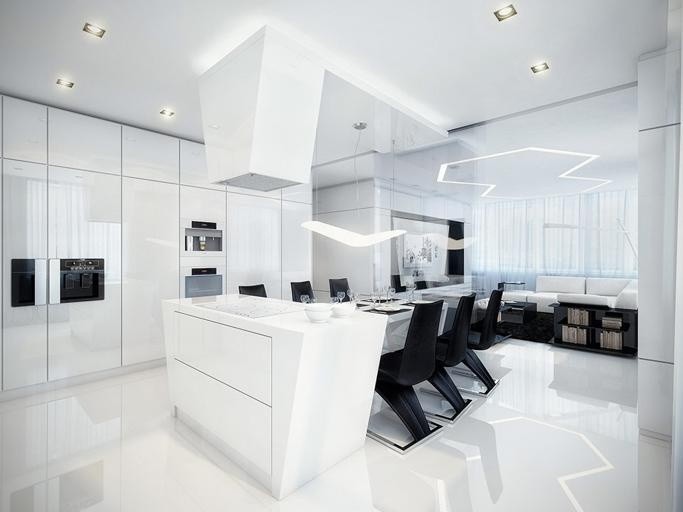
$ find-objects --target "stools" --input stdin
[501,290,533,304]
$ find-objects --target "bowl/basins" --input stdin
[329,304,355,318]
[304,303,331,323]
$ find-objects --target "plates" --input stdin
[360,307,399,312]
[411,300,434,305]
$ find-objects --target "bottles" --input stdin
[197,234,206,251]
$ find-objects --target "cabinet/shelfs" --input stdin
[162,294,387,500]
[548,303,638,358]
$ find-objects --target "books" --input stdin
[562,307,623,350]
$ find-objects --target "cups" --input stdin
[300,295,318,303]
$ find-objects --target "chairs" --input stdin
[289,280,316,302]
[369,298,446,456]
[454,289,501,399]
[418,291,475,426]
[327,278,352,297]
[238,283,269,298]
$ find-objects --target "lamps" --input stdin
[300,122,407,248]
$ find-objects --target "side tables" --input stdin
[501,302,537,325]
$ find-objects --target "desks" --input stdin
[498,283,525,290]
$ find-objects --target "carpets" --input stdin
[500,312,554,343]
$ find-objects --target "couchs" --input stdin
[527,276,638,310]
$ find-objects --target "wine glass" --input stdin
[336,284,418,307]
